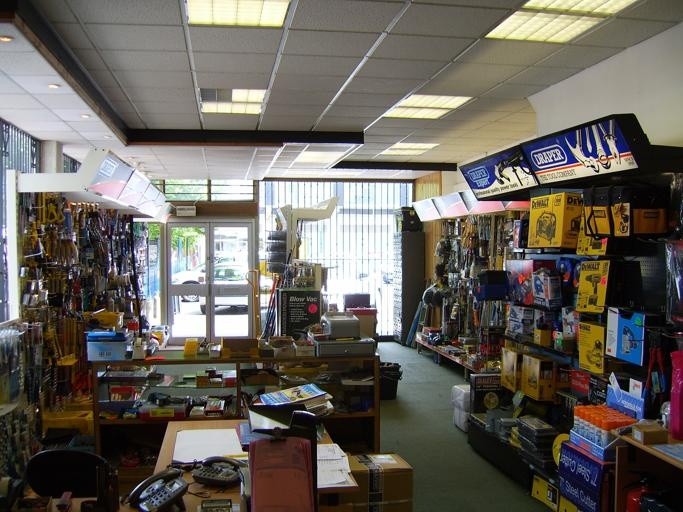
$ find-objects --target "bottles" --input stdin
[285,263,312,288]
[574,405,637,447]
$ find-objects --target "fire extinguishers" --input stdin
[623,476,656,512]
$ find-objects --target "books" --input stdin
[237,382,334,452]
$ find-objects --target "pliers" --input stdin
[642,328,666,405]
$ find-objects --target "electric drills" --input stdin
[585,273,601,305]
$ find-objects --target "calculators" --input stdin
[201,499,232,512]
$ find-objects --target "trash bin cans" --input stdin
[379,362,403,400]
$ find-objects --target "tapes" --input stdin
[136,338,142,347]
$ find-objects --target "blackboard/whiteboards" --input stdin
[279,289,322,342]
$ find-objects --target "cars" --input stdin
[176,251,273,314]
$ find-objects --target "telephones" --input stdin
[128,467,189,512]
[192,457,248,488]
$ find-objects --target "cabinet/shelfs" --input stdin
[92,355,380,497]
[450,207,521,373]
[501,196,665,509]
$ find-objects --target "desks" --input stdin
[612,421,683,512]
[0,419,252,511]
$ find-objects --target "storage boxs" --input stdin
[85,330,128,362]
[318,454,413,512]
